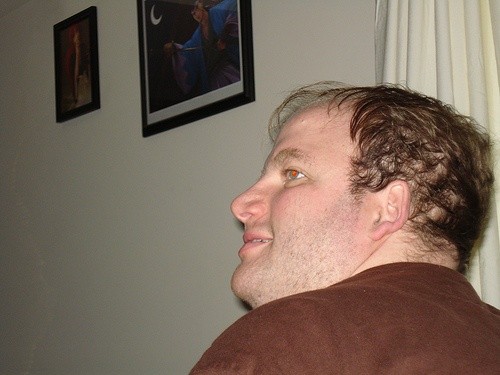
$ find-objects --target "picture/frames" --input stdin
[53,6,101,124]
[136,0,256,138]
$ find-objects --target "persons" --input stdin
[189,80,500,375]
